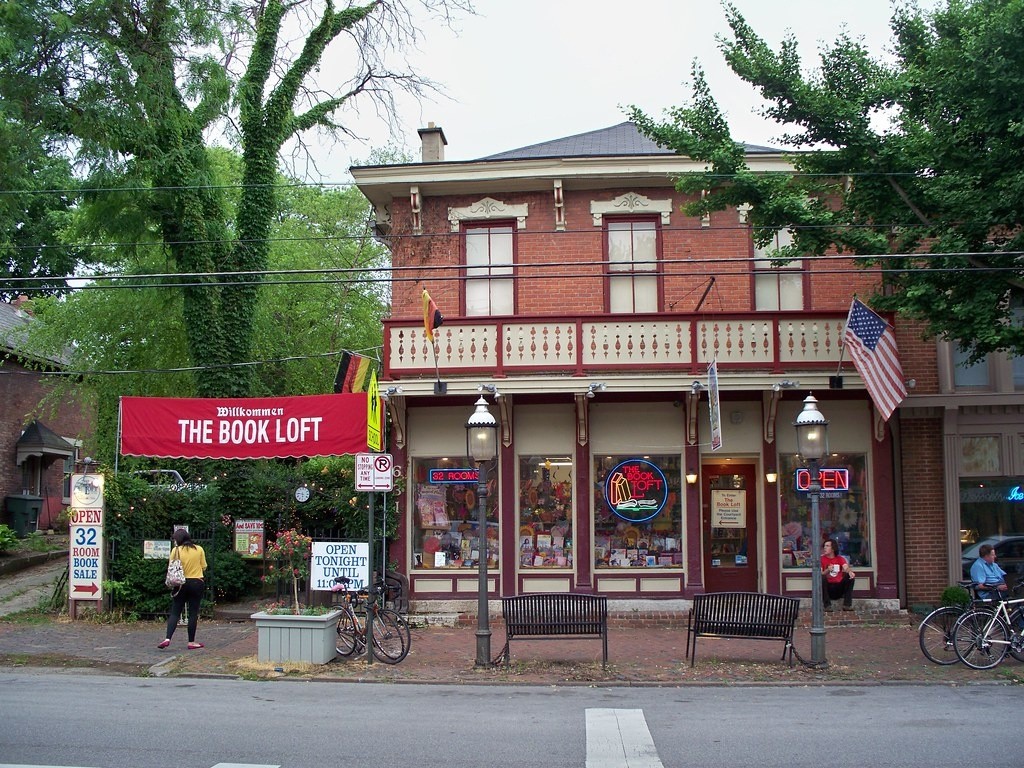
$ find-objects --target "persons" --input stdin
[157,529,207,649]
[820,539,855,611]
[971,544,1011,609]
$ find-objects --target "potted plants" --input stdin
[251,528,343,665]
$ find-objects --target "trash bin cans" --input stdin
[4,494,46,539]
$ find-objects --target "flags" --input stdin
[844,297,907,422]
[422,290,444,344]
[332,352,371,394]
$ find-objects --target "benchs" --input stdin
[686,592,800,667]
[501,594,609,671]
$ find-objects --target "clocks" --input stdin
[295,486,311,504]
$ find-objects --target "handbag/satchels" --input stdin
[165,546,185,586]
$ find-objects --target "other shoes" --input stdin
[823,606,833,612]
[158,640,170,649]
[843,606,854,610]
[188,643,204,649]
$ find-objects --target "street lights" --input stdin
[792,389,831,673]
[465,390,500,668]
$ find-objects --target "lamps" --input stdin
[772,383,779,391]
[691,379,703,394]
[586,385,595,398]
[783,380,800,389]
[488,384,502,398]
[395,385,403,393]
[477,383,486,391]
[594,381,606,392]
[381,386,396,401]
[905,379,916,388]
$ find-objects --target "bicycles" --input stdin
[334,575,413,667]
[917,577,1024,671]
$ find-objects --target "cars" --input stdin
[129,468,209,494]
[431,517,501,537]
[962,531,1024,579]
[801,520,862,555]
